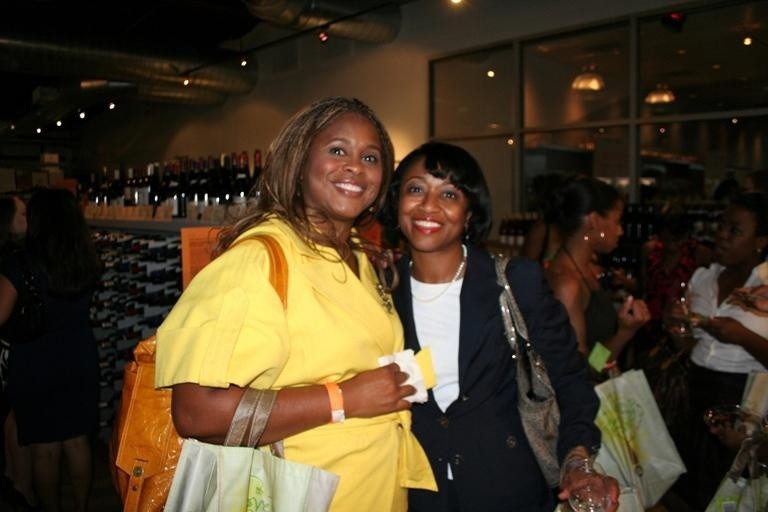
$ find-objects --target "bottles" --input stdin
[497,198,724,281]
[82,149,280,433]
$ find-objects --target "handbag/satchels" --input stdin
[161,386,338,512]
[704,438,768,512]
[591,365,688,512]
[494,253,563,491]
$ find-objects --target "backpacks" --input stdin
[109,234,289,512]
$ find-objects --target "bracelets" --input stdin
[323,382,346,425]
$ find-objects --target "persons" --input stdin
[685,279,768,378]
[661,186,767,453]
[514,174,570,261]
[1,182,106,510]
[541,172,651,387]
[154,93,427,510]
[376,138,624,511]
[1,192,31,488]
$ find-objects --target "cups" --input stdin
[563,461,610,512]
